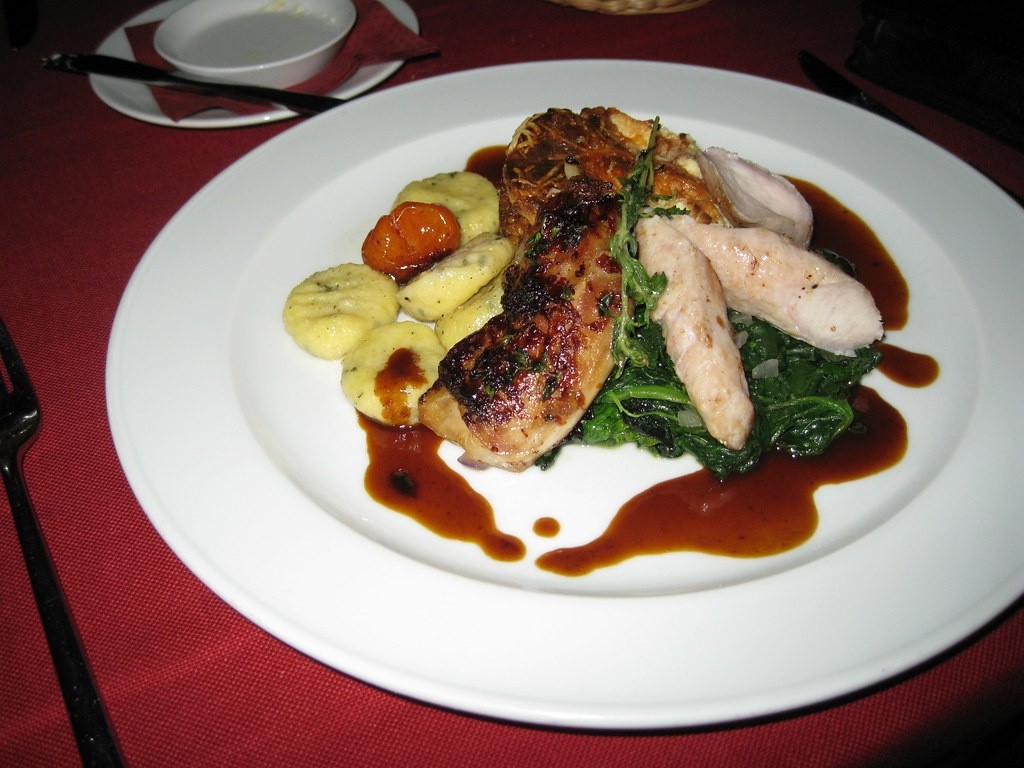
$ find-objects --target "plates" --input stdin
[104,59,1024,730]
[89,0,419,128]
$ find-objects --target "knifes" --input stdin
[796,49,1024,207]
[41,54,346,114]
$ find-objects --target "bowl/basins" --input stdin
[155,0,356,89]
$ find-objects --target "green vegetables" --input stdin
[529,116,881,481]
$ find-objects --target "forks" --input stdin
[0,316,129,768]
